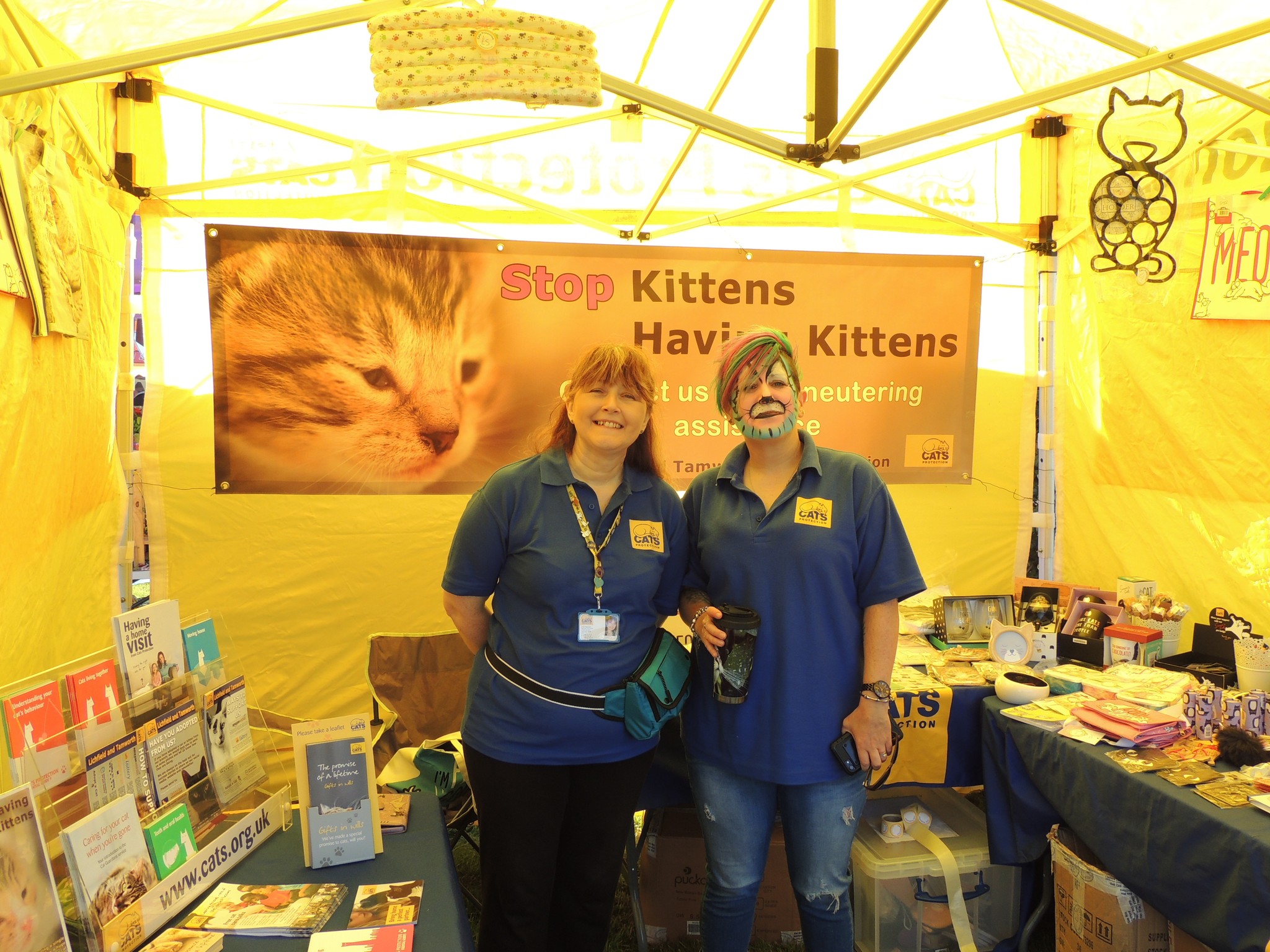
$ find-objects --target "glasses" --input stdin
[863,731,899,790]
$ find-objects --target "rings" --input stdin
[704,620,710,629]
[879,751,887,757]
[704,631,708,638]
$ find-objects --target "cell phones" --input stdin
[831,712,904,775]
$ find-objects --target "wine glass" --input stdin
[1024,593,1054,632]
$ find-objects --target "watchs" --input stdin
[861,680,891,699]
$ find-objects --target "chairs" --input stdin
[246,629,481,854]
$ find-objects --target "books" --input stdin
[308,924,415,952]
[347,879,424,929]
[0,598,349,952]
[378,794,411,835]
[291,712,384,868]
[306,736,375,870]
[890,598,1270,815]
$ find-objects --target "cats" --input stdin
[204,224,504,497]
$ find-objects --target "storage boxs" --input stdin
[1046,824,1217,952]
[849,787,1021,952]
[640,805,803,945]
[929,577,1263,711]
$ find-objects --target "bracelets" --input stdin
[860,693,889,703]
[690,606,708,643]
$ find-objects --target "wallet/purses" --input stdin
[1071,700,1193,748]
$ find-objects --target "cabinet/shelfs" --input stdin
[117,215,149,614]
[0,608,294,952]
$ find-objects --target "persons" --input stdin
[680,327,926,952]
[442,343,687,952]
[605,616,617,636]
[225,884,387,928]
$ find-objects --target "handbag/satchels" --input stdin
[379,733,479,823]
[626,628,696,741]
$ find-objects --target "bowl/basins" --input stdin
[995,664,1191,712]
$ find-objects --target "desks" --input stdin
[625,641,1058,951]
[69,792,477,952]
[980,696,1270,952]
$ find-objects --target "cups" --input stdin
[1110,636,1140,665]
[1223,698,1241,731]
[974,599,1003,640]
[1235,664,1270,693]
[1241,689,1270,736]
[1072,608,1114,640]
[944,599,974,641]
[711,602,760,704]
[1207,687,1222,737]
[1195,694,1212,740]
[1077,595,1106,606]
[1182,689,1199,736]
[1162,639,1180,657]
